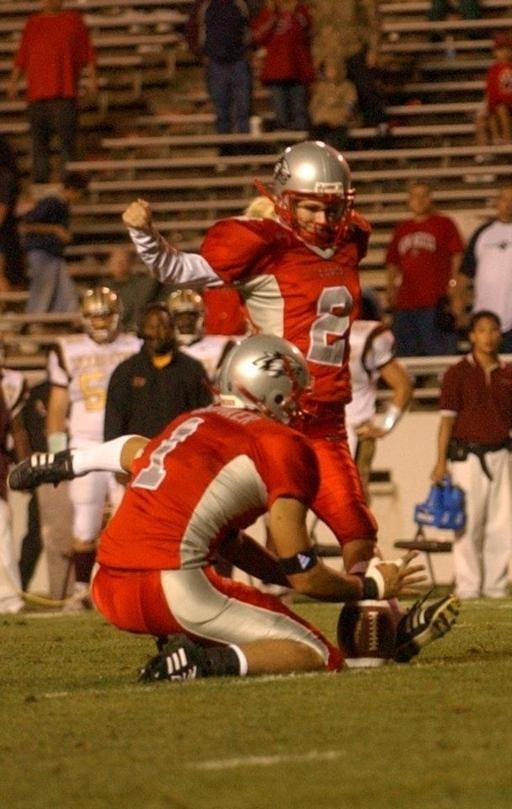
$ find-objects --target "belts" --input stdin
[466,441,508,455]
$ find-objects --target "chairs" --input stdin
[391,511,456,597]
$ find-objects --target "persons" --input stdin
[0,172,239,613]
[305,179,511,601]
[8,140,464,663]
[121,137,378,571]
[85,336,432,686]
[10,1,512,188]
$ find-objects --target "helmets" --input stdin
[80,287,124,344]
[167,289,205,347]
[214,333,318,428]
[255,140,357,249]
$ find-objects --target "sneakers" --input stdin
[63,590,89,613]
[133,633,211,683]
[6,448,88,491]
[0,599,37,613]
[393,584,460,663]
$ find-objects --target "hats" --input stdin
[494,31,512,48]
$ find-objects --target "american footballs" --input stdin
[337,600,395,668]
